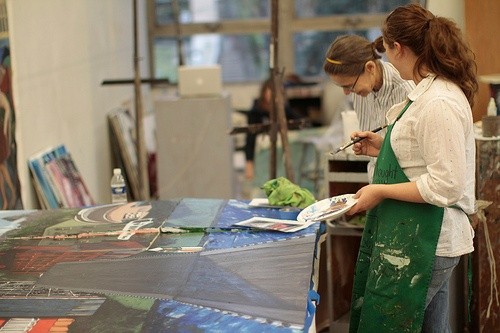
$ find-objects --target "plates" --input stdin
[296,193,359,222]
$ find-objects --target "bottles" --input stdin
[110,168,128,204]
[487,97,497,116]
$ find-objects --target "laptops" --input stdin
[176,64,222,97]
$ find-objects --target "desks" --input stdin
[153,92,236,199]
[0,198,322,333]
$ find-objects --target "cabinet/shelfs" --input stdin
[323,148,484,323]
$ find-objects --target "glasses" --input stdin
[349,73,361,94]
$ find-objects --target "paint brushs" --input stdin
[330,124,387,155]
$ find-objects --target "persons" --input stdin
[244,73,316,181]
[321,33,416,185]
[346,2,478,333]
